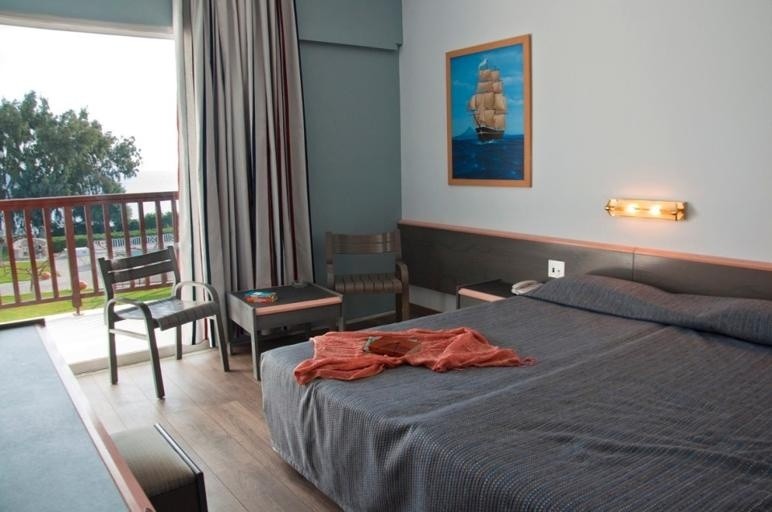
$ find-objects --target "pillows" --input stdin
[535,273,772,341]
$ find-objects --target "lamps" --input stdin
[605,199,689,222]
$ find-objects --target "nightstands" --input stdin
[456,279,528,311]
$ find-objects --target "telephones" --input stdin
[511,280,544,296]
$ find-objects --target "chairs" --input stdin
[323,229,414,338]
[97,249,230,401]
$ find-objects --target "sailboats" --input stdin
[467,66,508,142]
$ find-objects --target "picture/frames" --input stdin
[444,33,533,190]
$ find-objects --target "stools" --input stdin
[109,424,209,512]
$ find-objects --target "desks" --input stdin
[0,315,156,511]
[225,279,358,381]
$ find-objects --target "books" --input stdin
[244,288,279,306]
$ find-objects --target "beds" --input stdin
[260,268,772,512]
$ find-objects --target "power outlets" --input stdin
[547,259,565,279]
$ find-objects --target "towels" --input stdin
[293,325,539,386]
